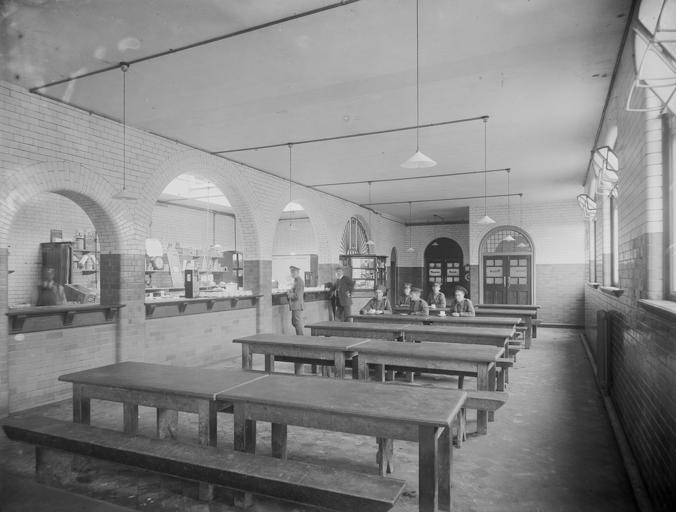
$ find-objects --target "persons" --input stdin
[450,284,475,316]
[408,287,430,316]
[427,281,446,308]
[327,267,356,320]
[398,282,414,305]
[359,284,392,316]
[287,266,305,334]
[30,265,68,304]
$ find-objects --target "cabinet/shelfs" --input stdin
[339,253,377,291]
[40,242,230,306]
[376,256,388,291]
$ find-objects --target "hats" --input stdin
[289,265,300,270]
[374,285,386,294]
[455,285,469,295]
[411,286,423,292]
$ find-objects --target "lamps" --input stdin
[398,0,438,169]
[279,139,530,255]
[475,115,497,225]
[110,61,139,202]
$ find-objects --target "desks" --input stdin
[0,473,144,511]
[229,301,540,445]
[55,360,271,502]
[216,375,469,510]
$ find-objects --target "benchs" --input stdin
[2,414,408,511]
